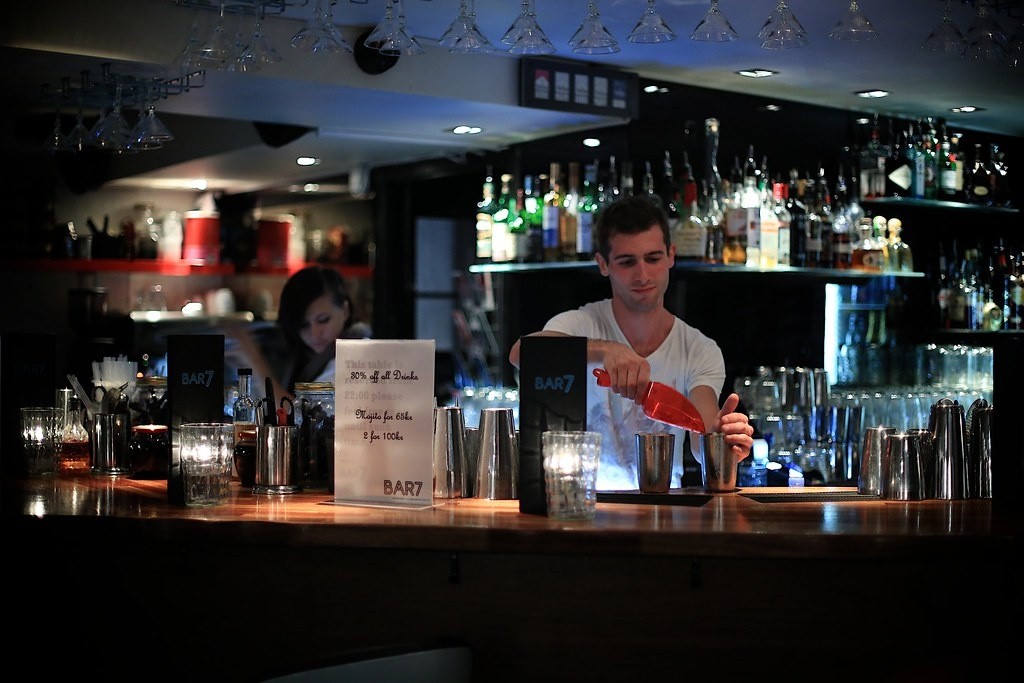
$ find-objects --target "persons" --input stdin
[508,197,754,492]
[224,268,371,426]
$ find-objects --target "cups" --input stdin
[177,422,234,509]
[55,389,75,408]
[471,408,520,499]
[735,343,862,486]
[19,407,65,475]
[181,302,204,318]
[432,406,471,498]
[256,214,296,269]
[252,423,304,495]
[698,432,739,492]
[856,424,934,501]
[90,412,132,475]
[634,431,676,494]
[182,210,220,261]
[91,385,130,412]
[541,430,601,519]
[863,342,994,430]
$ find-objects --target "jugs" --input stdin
[927,396,993,501]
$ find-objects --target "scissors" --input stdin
[256,396,294,428]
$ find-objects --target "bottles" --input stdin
[232,367,257,423]
[126,375,168,428]
[129,424,169,479]
[566,1,623,57]
[60,396,90,462]
[472,109,1023,340]
[91,285,110,316]
[234,430,257,488]
[161,209,182,260]
[285,381,335,494]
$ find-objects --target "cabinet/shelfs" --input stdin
[469,194,1024,337]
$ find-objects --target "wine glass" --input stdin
[437,0,498,57]
[289,0,355,57]
[824,0,882,44]
[919,0,1024,73]
[40,78,176,155]
[689,0,741,44]
[363,1,429,56]
[626,1,678,44]
[757,0,812,51]
[500,0,557,56]
[168,0,285,74]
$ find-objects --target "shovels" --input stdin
[591,367,706,436]
